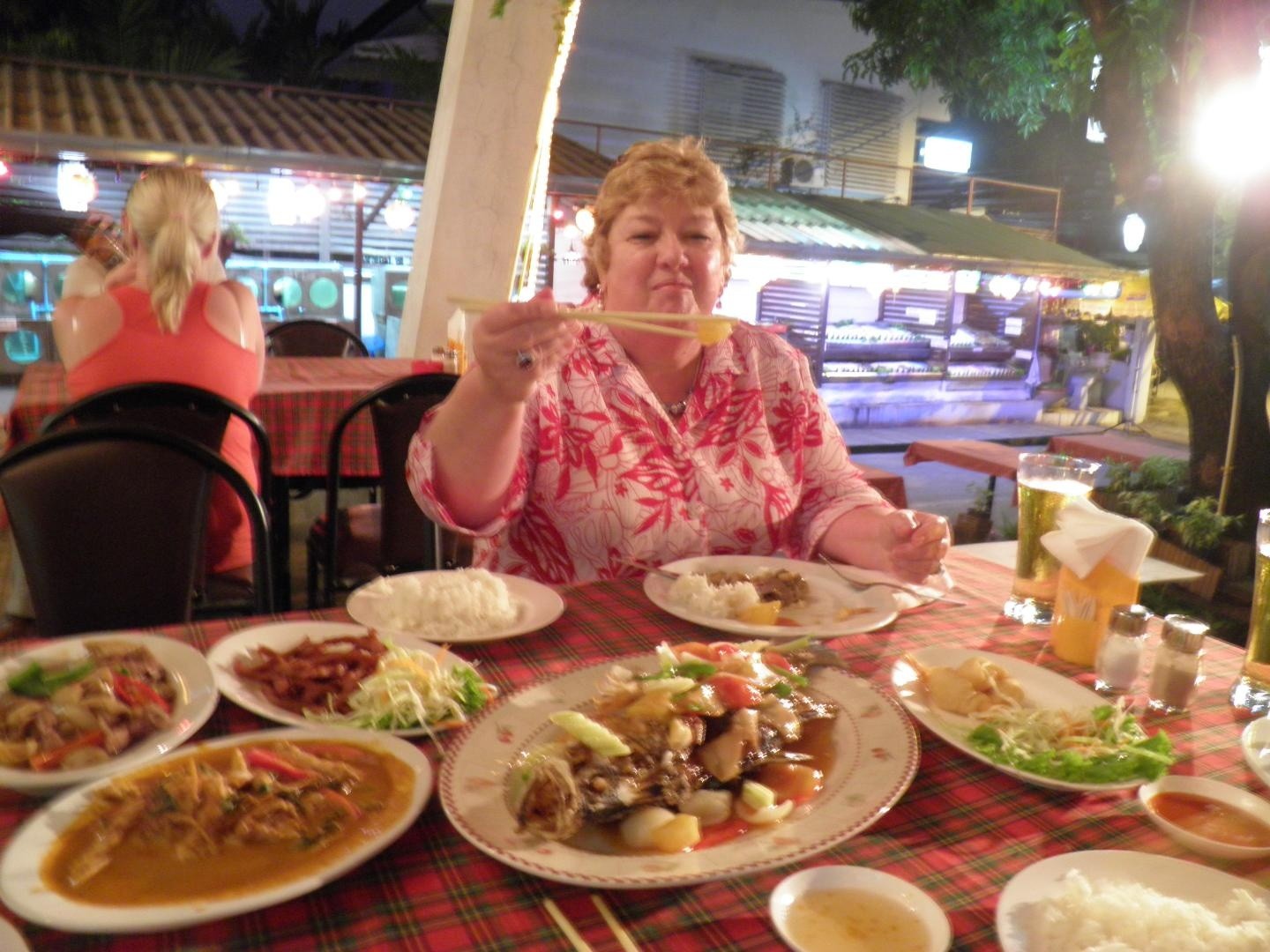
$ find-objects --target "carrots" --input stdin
[110,673,171,712]
[27,729,104,770]
[248,750,304,778]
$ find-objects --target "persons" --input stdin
[48,161,271,623]
[402,132,954,585]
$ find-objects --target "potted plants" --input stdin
[958,479,1000,543]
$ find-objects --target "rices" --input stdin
[1024,869,1270,952]
[669,570,762,617]
[355,565,518,636]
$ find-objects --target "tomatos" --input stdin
[706,641,791,708]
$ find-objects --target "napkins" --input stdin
[1037,491,1155,576]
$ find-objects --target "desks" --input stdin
[0,358,443,610]
[903,440,1026,514]
[1044,430,1187,472]
[850,459,907,511]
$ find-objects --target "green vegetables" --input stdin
[638,659,810,711]
[8,659,131,695]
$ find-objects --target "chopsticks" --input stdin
[541,895,640,952]
[445,296,726,338]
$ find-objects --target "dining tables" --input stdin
[0,551,1269,952]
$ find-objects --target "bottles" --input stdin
[431,307,466,375]
[1094,603,1155,695]
[1146,613,1209,716]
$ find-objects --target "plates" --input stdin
[996,849,1269,952]
[206,620,486,737]
[890,644,1168,793]
[1137,774,1269,859]
[0,917,29,952]
[768,864,952,952]
[0,633,220,798]
[345,569,565,643]
[438,651,922,889]
[642,554,901,639]
[1241,715,1270,785]
[0,724,434,935]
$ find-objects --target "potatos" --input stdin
[694,317,733,345]
[746,598,780,625]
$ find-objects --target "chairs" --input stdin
[0,320,462,642]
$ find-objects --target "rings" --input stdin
[517,349,536,373]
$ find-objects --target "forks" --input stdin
[817,549,968,607]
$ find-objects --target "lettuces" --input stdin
[352,663,488,730]
[967,706,1177,783]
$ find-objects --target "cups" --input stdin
[1048,555,1139,671]
[1004,453,1102,626]
[1228,508,1270,714]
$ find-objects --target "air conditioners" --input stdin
[785,155,826,190]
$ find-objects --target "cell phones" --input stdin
[74,222,130,271]
